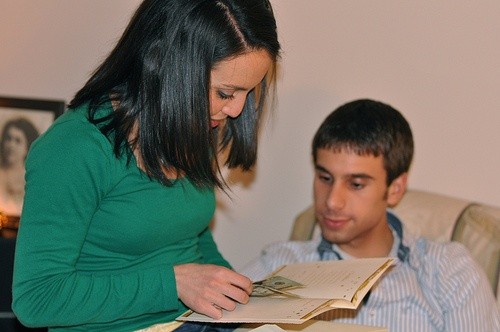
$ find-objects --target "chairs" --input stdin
[290,189,500,300]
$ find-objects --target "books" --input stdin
[175,258,394,324]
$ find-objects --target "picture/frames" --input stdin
[0,95,67,217]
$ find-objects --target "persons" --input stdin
[11,0,283,332]
[240,98,499,332]
